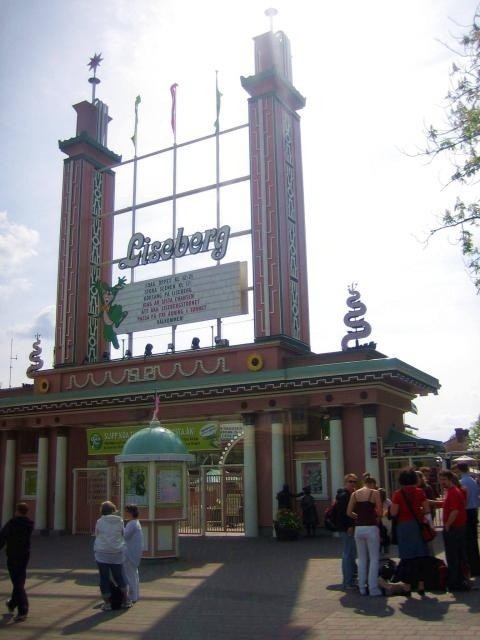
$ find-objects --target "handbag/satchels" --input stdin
[420,513,438,543]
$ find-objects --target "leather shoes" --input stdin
[416,589,425,595]
[396,589,410,597]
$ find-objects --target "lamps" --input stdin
[71,334,229,365]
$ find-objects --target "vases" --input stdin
[274,527,307,544]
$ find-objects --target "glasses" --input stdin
[346,480,357,484]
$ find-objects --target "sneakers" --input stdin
[359,589,367,596]
[14,615,27,622]
[368,588,384,596]
[101,603,112,611]
[121,600,134,608]
[5,599,16,613]
[344,585,358,590]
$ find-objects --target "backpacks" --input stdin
[323,502,344,534]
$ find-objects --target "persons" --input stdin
[325,462,480,596]
[94,501,133,610]
[0,501,35,622]
[276,484,304,510]
[123,504,144,603]
[298,485,321,537]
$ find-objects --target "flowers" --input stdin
[272,506,305,534]
